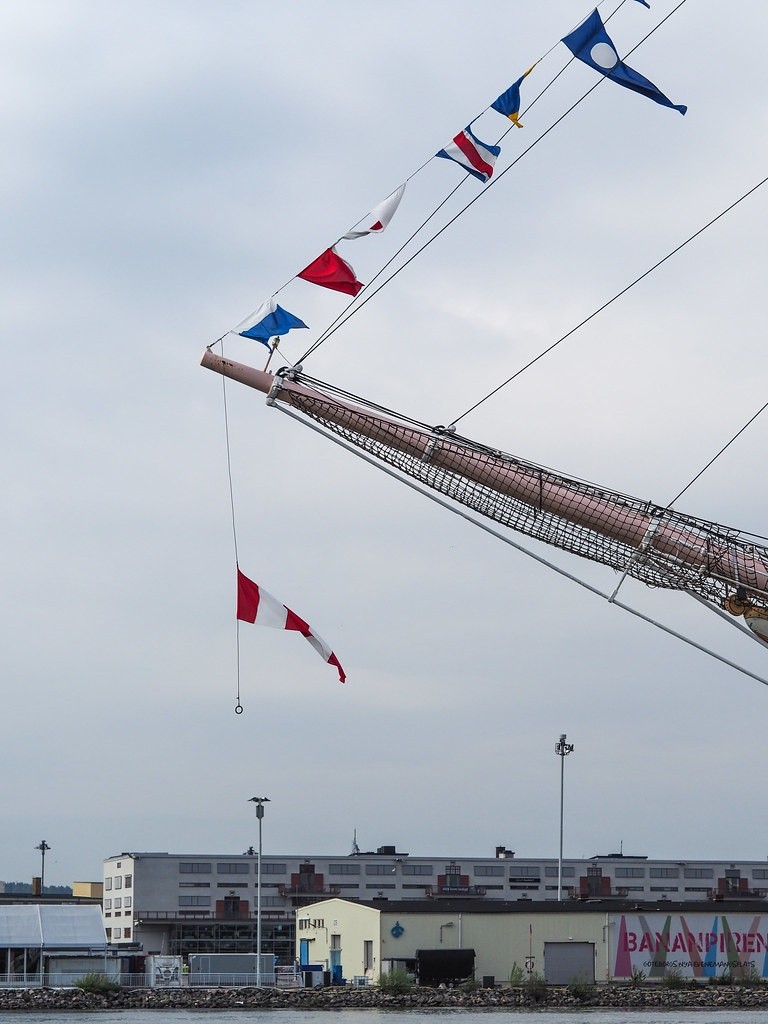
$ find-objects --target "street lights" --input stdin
[554,734,574,901]
[248,795,272,986]
[34,840,51,894]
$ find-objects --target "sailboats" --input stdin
[200,2,768,703]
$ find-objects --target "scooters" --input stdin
[153,955,183,987]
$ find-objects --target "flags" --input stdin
[340,181,407,240]
[296,245,365,298]
[238,571,347,684]
[560,8,688,117]
[230,297,310,351]
[490,66,525,128]
[434,124,502,184]
[634,0,651,9]
